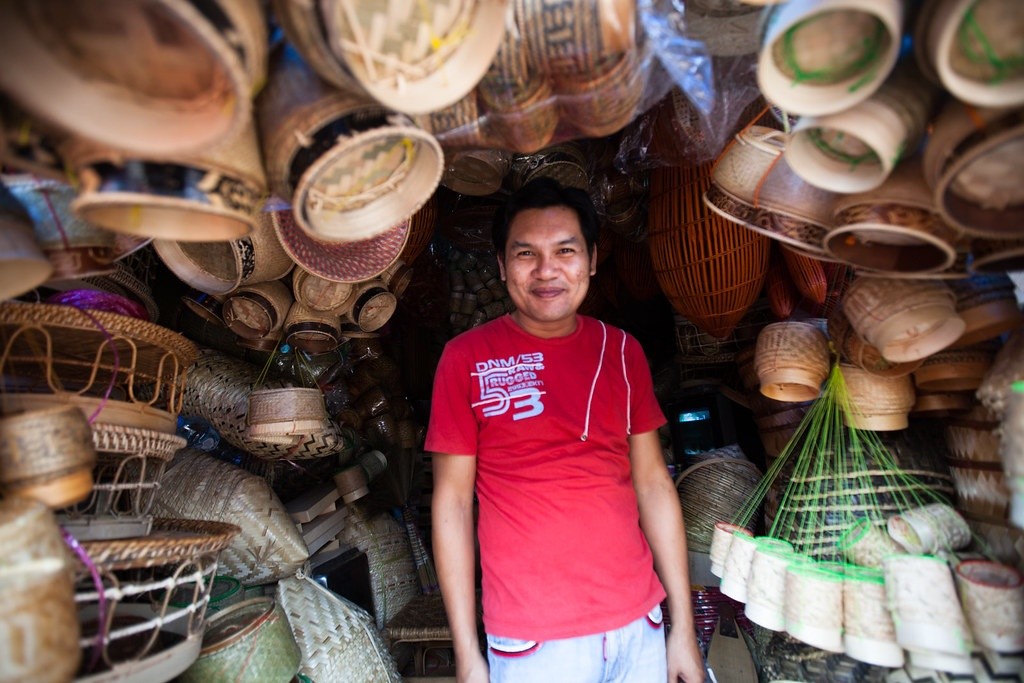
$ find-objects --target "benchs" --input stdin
[382,596,487,683]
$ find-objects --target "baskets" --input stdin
[0,0,1024,683]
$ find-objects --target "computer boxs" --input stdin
[668,390,741,472]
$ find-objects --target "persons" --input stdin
[424,175,706,683]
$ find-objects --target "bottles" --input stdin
[269,346,300,378]
[179,414,244,466]
